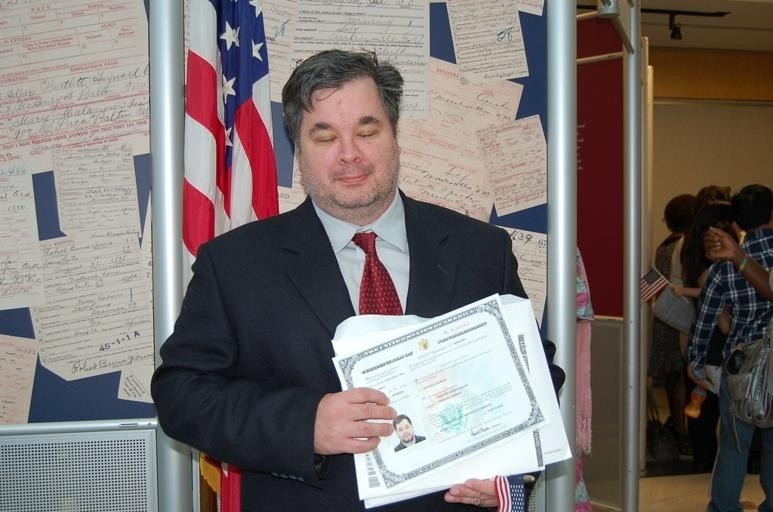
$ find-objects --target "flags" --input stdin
[183,0,273,509]
[638,269,667,303]
[497,474,525,512]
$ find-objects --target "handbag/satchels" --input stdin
[723,322,773,427]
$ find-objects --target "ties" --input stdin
[351,231,401,314]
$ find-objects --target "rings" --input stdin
[476,499,485,507]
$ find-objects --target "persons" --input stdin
[392,413,426,452]
[685,182,772,512]
[650,184,772,474]
[150,46,566,511]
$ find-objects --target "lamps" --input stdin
[666,13,685,40]
